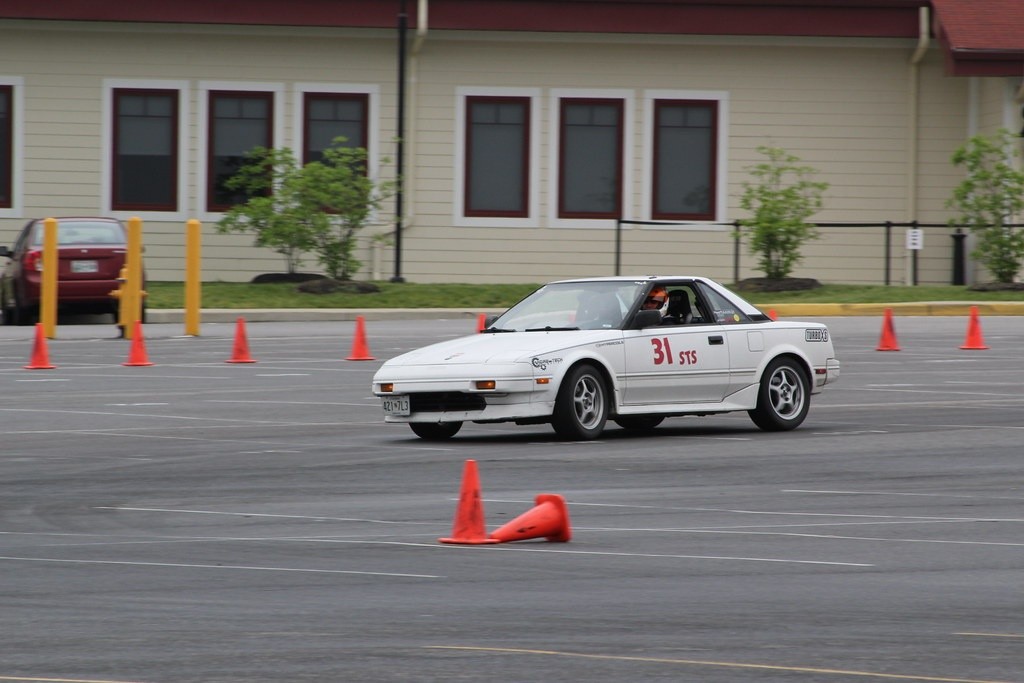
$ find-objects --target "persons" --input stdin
[634,285,684,321]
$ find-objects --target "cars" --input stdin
[0,214,149,324]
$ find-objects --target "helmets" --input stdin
[634,284,669,318]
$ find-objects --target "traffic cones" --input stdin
[490,493,573,546]
[225,316,259,363]
[475,312,487,334]
[345,315,378,361]
[23,323,58,369]
[959,305,990,349]
[875,307,901,352]
[119,319,154,367]
[769,308,776,321]
[435,458,501,546]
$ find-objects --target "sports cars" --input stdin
[370,276,842,440]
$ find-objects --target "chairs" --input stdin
[668,290,694,324]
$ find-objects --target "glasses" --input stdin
[643,300,657,304]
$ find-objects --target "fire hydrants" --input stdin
[108,266,150,336]
